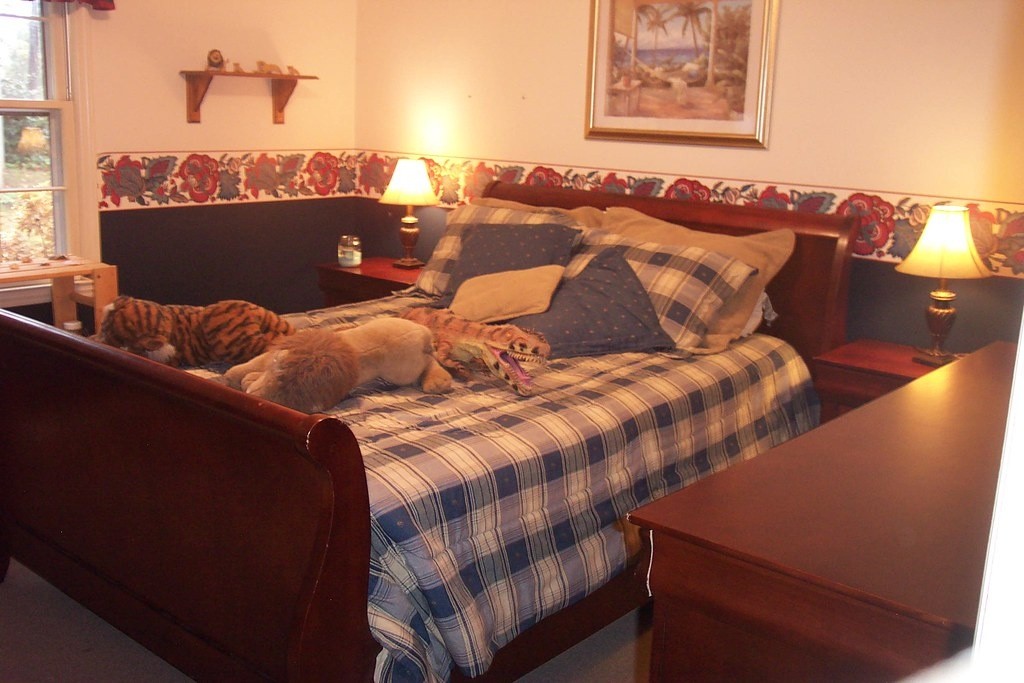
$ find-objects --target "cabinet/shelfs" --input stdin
[621,337,1022,683]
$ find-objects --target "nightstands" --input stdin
[806,337,966,427]
[313,256,426,310]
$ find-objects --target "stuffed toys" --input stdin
[226,318,450,412]
[393,309,549,397]
[97,296,295,370]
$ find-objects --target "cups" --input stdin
[338,236,362,267]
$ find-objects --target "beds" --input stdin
[0,177,862,683]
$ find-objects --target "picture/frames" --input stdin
[584,0,781,151]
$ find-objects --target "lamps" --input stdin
[892,203,1002,365]
[377,158,443,270]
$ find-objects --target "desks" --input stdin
[0,253,119,338]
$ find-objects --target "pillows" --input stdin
[419,222,582,311]
[602,205,796,357]
[506,247,680,359]
[470,198,604,235]
[446,264,564,323]
